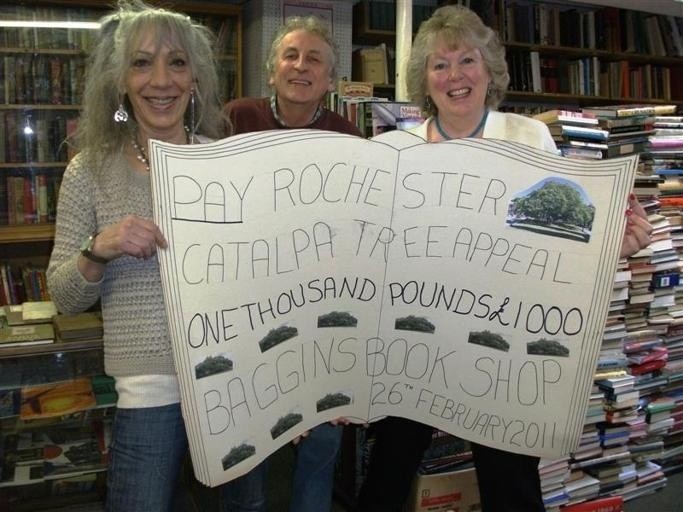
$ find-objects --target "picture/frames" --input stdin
[280,0,334,41]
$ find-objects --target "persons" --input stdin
[345,3,654,512]
[209,15,367,511]
[44,2,264,512]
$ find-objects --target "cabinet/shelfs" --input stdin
[352,0,681,114]
[1,0,248,511]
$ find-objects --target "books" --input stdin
[532,102,683,512]
[418,2,682,116]
[1,0,109,226]
[1,251,118,512]
[321,1,419,142]
[189,12,238,105]
[419,429,475,475]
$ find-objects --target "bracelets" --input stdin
[129,122,193,171]
